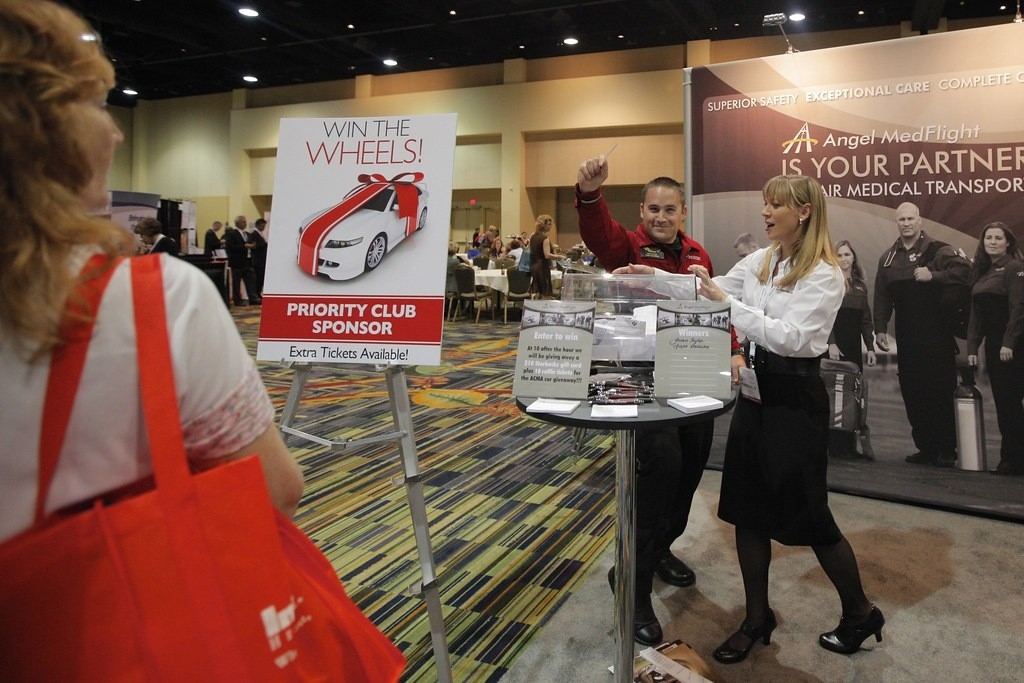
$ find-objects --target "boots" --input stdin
[859,426,875,461]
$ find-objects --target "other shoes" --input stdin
[906,450,937,464]
[938,452,956,468]
[990,465,1023,475]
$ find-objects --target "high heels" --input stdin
[819,604,886,654]
[714,608,777,665]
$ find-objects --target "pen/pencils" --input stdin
[586,377,657,404]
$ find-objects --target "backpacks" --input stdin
[924,240,976,339]
[818,357,862,433]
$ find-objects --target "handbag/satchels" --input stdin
[0,255,407,683]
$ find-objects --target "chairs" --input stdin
[443,256,532,327]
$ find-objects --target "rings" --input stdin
[705,279,710,285]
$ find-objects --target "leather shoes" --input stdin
[653,550,696,586]
[632,620,663,645]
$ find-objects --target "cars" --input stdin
[294,180,430,281]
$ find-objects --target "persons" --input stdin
[0,0,305,543]
[575,155,746,646]
[734,233,759,258]
[133,217,176,257]
[874,202,971,467]
[204,215,268,306]
[613,174,884,663]
[447,215,603,315]
[824,240,877,461]
[967,222,1024,474]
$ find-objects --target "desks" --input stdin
[473,267,562,318]
[515,385,735,683]
[466,258,500,270]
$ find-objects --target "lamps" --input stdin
[762,12,800,53]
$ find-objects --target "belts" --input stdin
[744,342,821,376]
